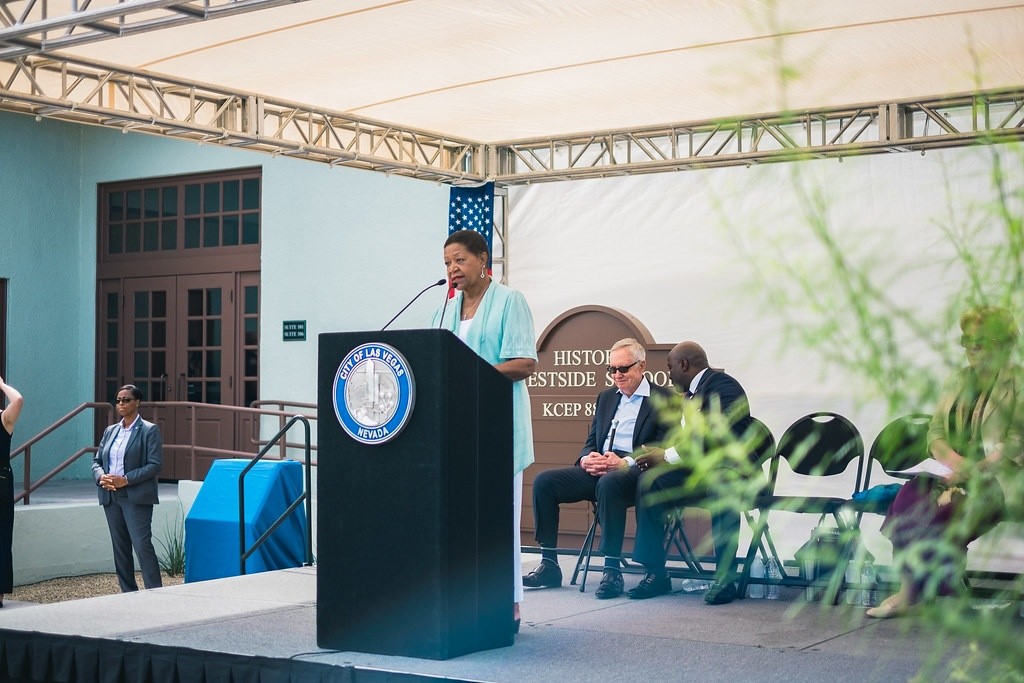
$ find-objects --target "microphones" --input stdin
[382,279,446,330]
[439,282,458,329]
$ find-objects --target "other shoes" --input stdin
[514,617,520,634]
[866,591,926,618]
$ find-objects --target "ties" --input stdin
[684,389,692,406]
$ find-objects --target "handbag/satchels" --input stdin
[794,505,875,587]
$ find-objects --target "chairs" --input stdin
[832,414,935,606]
[659,415,776,598]
[570,429,700,592]
[738,412,881,605]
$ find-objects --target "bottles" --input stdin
[749,555,764,598]
[682,578,716,591]
[845,560,861,604]
[861,561,877,606]
[765,556,779,599]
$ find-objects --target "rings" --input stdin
[644,462,648,468]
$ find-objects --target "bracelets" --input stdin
[123,474,130,486]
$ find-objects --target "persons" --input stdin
[0,377,23,597]
[864,304,1024,618]
[90,385,164,592]
[519,338,749,604]
[430,228,538,634]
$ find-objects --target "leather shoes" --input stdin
[627,572,672,599]
[705,580,736,605]
[595,572,624,598]
[522,564,561,588]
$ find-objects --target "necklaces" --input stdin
[462,276,493,320]
[972,393,982,403]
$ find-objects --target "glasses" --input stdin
[606,360,640,374]
[115,399,137,404]
[960,333,1008,350]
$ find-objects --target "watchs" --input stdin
[624,460,630,469]
